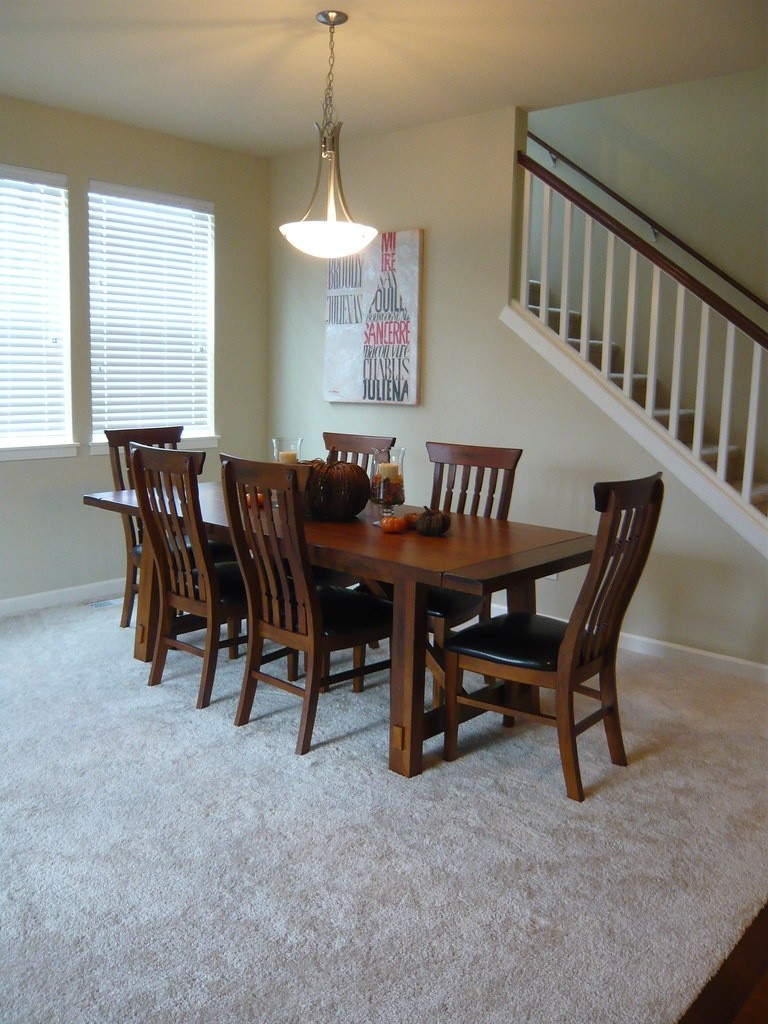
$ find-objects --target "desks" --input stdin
[82,481,620,781]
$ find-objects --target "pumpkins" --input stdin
[382,505,452,536]
[246,492,264,507]
[297,446,370,519]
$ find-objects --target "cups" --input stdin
[271,437,304,466]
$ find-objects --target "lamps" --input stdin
[279,11,379,259]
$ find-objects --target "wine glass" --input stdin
[368,448,406,528]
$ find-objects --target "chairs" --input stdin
[102,423,243,634]
[302,432,396,672]
[219,451,394,754]
[351,442,523,711]
[127,440,299,709]
[444,471,665,803]
